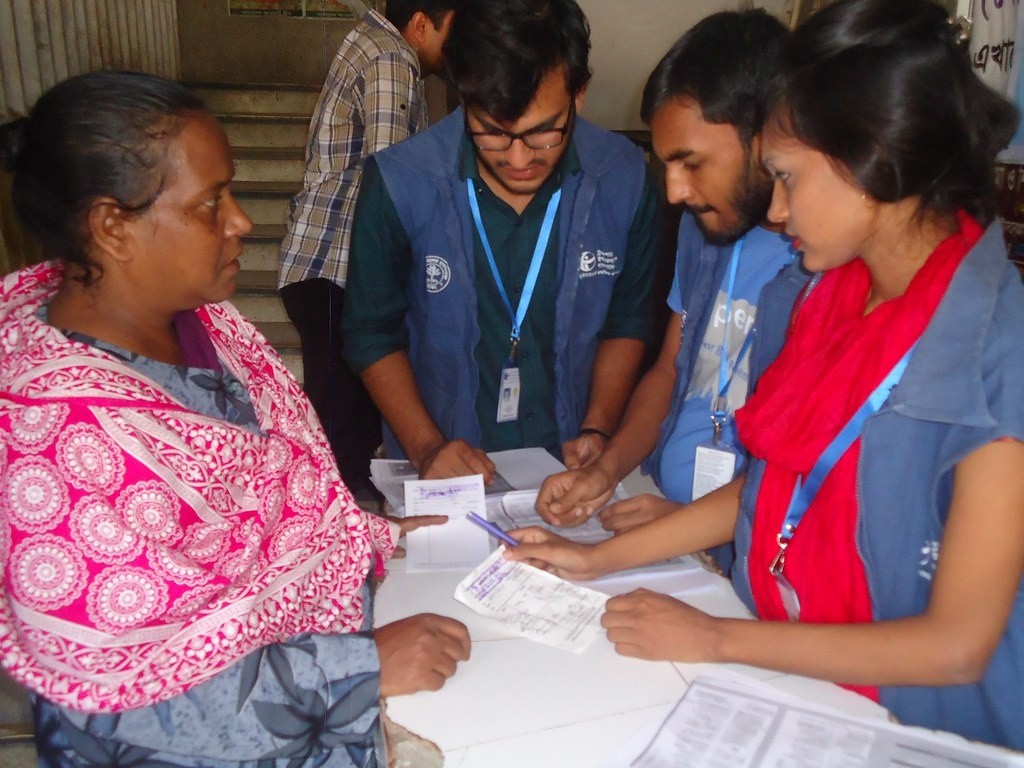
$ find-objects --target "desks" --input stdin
[371,465,903,768]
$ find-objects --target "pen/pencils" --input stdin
[465,510,520,547]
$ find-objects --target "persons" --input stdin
[0,67,472,768]
[276,0,455,510]
[500,0,1024,755]
[534,0,822,582]
[338,0,681,488]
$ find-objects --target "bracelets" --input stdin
[577,428,611,442]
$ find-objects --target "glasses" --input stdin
[463,91,573,152]
